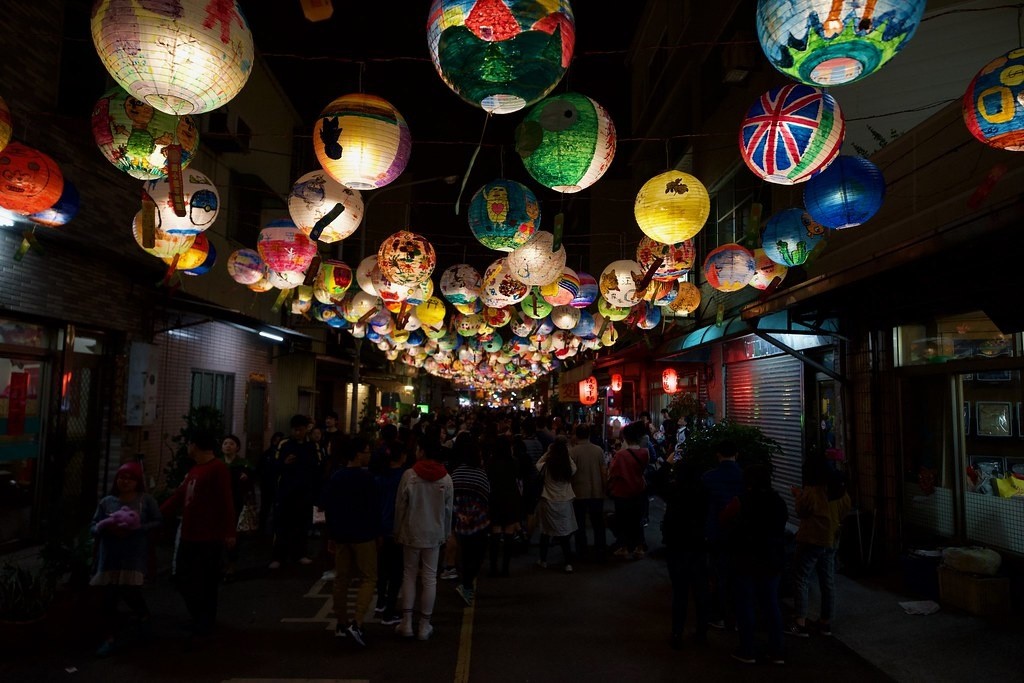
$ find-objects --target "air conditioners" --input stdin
[720,31,762,83]
[202,112,252,152]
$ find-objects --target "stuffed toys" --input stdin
[96,505,143,534]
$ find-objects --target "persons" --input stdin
[91,456,162,659]
[730,463,788,663]
[702,437,749,635]
[224,399,693,641]
[152,421,238,649]
[657,442,718,650]
[783,449,844,638]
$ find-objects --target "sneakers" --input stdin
[456,584,472,606]
[440,567,458,579]
[783,621,809,637]
[335,623,349,637]
[346,620,369,647]
[813,618,832,635]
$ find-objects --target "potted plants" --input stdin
[38,528,96,611]
[0,560,56,646]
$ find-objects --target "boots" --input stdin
[489,541,500,576]
[500,543,512,577]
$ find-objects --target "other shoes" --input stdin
[731,649,757,663]
[381,612,402,624]
[560,559,573,572]
[537,559,546,567]
[97,639,116,656]
[419,624,432,639]
[706,613,726,629]
[670,634,683,649]
[395,624,414,636]
[692,630,706,647]
[762,652,785,663]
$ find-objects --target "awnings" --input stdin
[651,305,851,385]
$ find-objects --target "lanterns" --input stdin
[579,375,598,405]
[611,372,623,392]
[0,0,934,393]
[963,47,1024,153]
[663,368,678,394]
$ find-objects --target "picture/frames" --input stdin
[954,339,1024,494]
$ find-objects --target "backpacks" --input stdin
[616,448,664,498]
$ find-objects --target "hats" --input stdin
[117,460,142,480]
